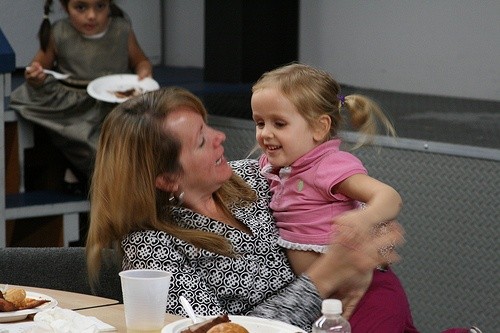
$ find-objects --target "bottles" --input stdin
[313,299,352,333]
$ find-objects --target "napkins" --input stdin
[34,308,116,332]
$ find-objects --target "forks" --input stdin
[26,67,72,80]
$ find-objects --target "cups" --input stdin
[119,269,172,333]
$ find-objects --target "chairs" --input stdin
[0,247,125,303]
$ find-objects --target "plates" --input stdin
[162,315,309,333]
[0,292,58,323]
[87,73,161,104]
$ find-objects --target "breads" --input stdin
[0,288,51,312]
[178,313,232,333]
[206,322,249,333]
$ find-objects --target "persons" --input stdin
[251,63,482,333]
[89,85,407,333]
[14,0,152,196]
